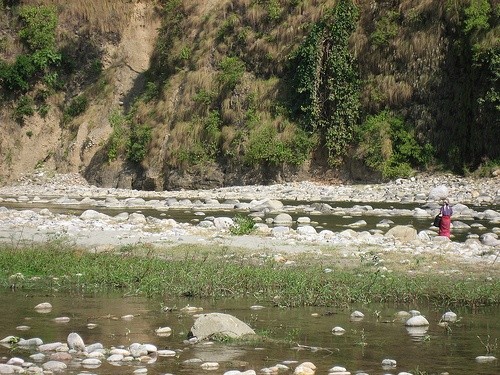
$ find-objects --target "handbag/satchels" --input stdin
[434,214,443,225]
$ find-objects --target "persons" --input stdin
[438,199,453,239]
